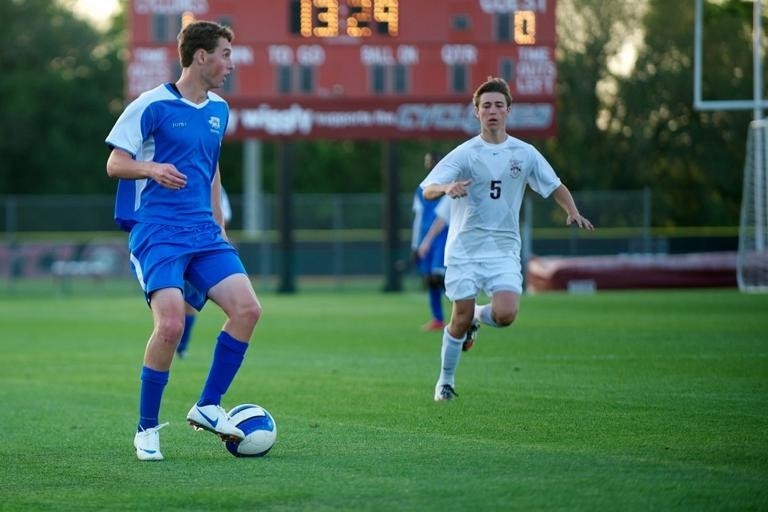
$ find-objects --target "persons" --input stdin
[409,152,464,334]
[418,77,595,401]
[111,136,234,364]
[416,181,456,268]
[98,20,262,463]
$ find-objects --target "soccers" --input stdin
[224,403,277,457]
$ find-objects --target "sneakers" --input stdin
[186,401,246,444]
[134,421,170,460]
[434,380,460,401]
[420,319,445,331]
[461,317,481,351]
